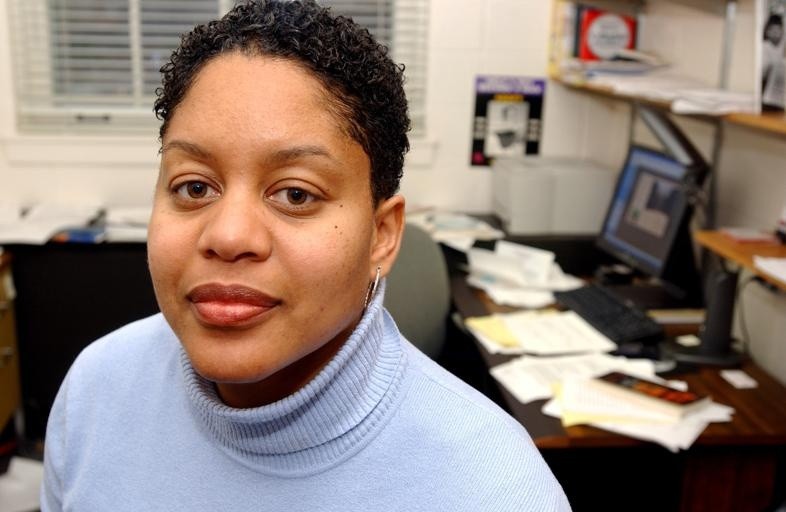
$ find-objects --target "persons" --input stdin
[41,1,572,512]
[762,14,785,99]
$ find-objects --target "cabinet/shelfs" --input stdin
[2,242,161,444]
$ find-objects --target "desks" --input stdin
[439,231,786,512]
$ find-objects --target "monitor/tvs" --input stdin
[594,143,706,285]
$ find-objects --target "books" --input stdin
[49,229,104,244]
[591,370,711,418]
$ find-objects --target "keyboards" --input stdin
[552,283,664,344]
